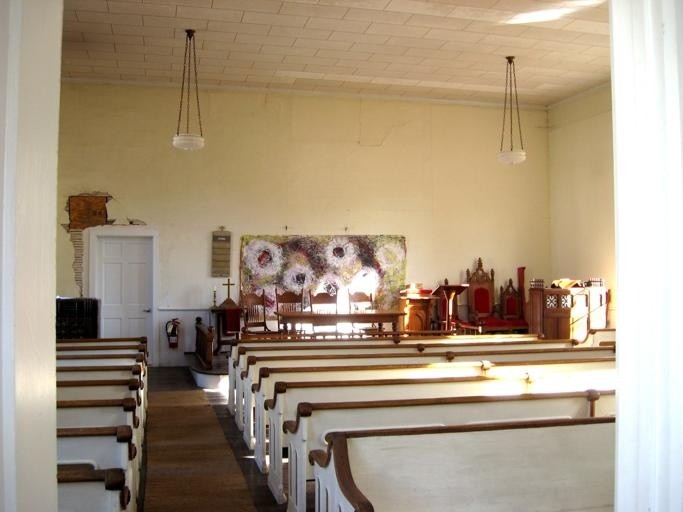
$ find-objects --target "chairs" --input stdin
[466,258,530,336]
[237,288,375,341]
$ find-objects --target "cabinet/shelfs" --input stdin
[528,278,611,346]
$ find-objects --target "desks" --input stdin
[273,310,408,344]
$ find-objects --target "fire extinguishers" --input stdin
[166,317,181,348]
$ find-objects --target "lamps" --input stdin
[172,23,205,150]
[494,54,528,166]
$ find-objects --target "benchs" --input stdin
[57,336,151,512]
[222,331,615,511]
